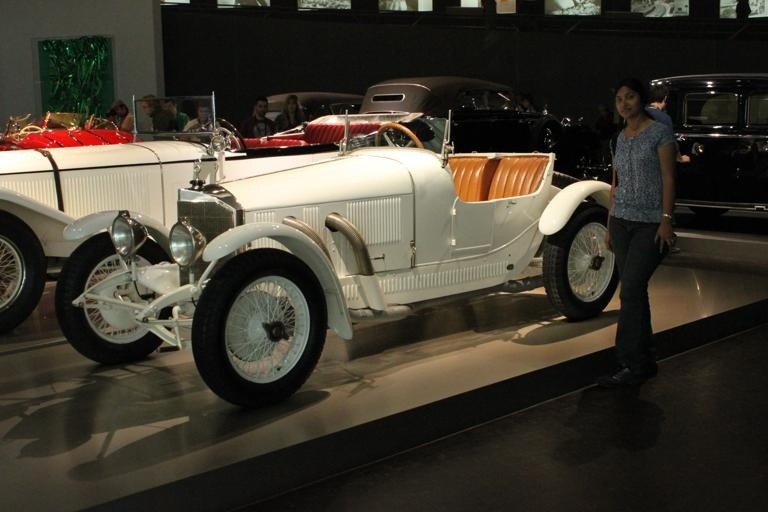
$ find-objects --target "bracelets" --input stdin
[659,212,673,221]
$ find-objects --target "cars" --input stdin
[54,107,621,410]
[647,71,768,217]
[0,91,424,340]
[251,92,365,133]
[360,75,587,155]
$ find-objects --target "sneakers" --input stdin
[599,361,657,391]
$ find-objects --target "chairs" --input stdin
[302,124,380,146]
[449,156,550,203]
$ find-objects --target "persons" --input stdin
[273,94,305,135]
[594,72,679,391]
[157,96,191,130]
[244,95,276,138]
[104,99,136,133]
[637,84,674,133]
[135,94,179,141]
[177,99,213,136]
